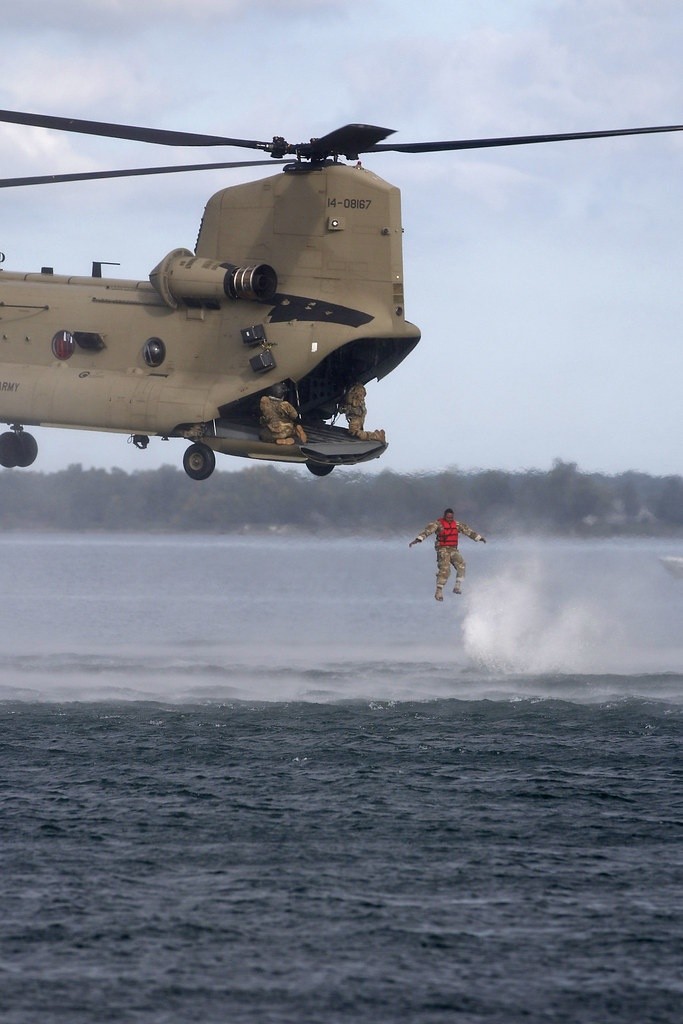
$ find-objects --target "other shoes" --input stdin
[296,425,307,443]
[374,429,386,445]
[276,438,295,445]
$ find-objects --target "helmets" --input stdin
[272,383,286,398]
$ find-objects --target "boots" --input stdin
[435,587,443,601]
[453,582,461,594]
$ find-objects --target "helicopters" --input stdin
[0,98,682,481]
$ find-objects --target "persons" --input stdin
[338,375,388,447]
[409,509,487,601]
[258,382,307,445]
